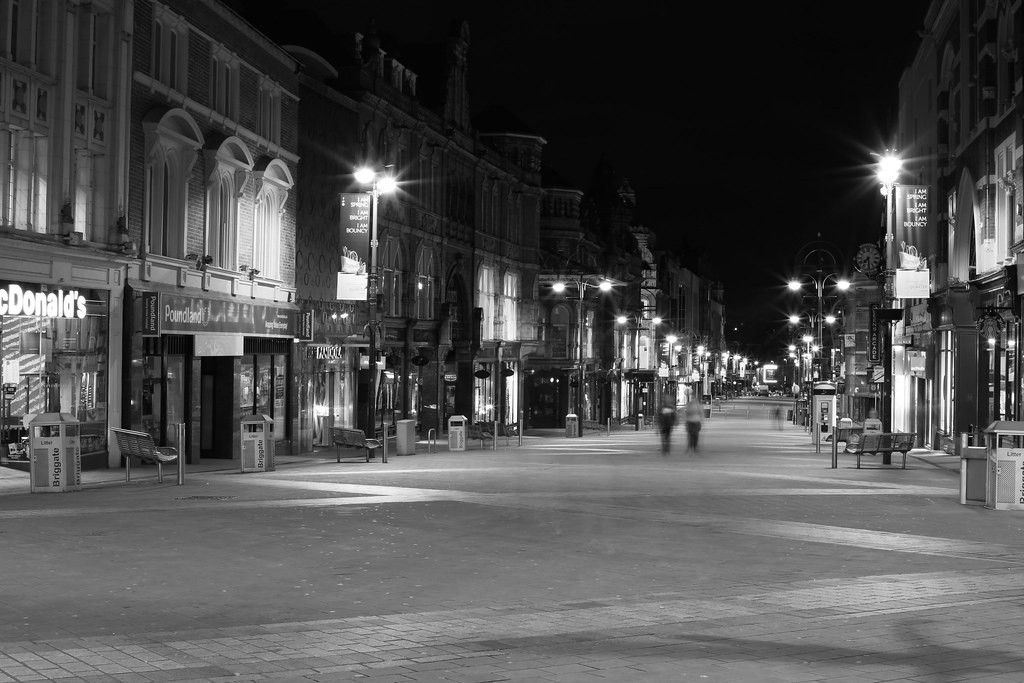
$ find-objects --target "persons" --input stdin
[684,398,704,452]
[658,394,677,456]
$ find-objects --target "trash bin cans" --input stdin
[840,417,852,430]
[637,414,644,431]
[864,419,882,437]
[566,413,579,437]
[240,414,275,472]
[448,415,469,452]
[787,410,793,421]
[715,398,720,406]
[29,412,82,494]
[983,420,1024,511]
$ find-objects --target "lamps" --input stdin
[569,376,578,388]
[387,349,403,370]
[474,362,490,379]
[499,361,514,377]
[411,348,430,366]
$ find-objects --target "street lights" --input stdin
[549,232,616,437]
[786,230,857,434]
[349,158,398,459]
[616,269,760,431]
[868,142,904,465]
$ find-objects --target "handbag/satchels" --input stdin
[340,246,360,273]
[898,245,920,270]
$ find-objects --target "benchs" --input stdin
[824,427,878,443]
[474,421,512,445]
[468,425,492,449]
[846,433,919,469]
[110,426,177,484]
[328,425,383,463]
